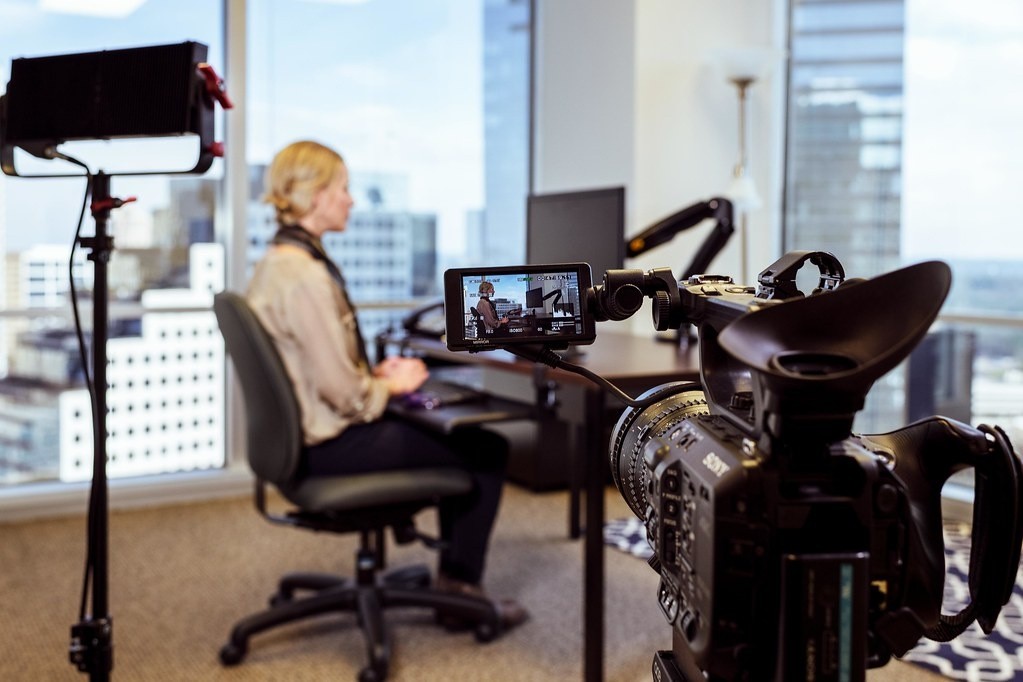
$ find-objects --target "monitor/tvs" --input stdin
[525,181,626,284]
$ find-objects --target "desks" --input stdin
[380,329,708,682]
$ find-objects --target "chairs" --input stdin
[214,293,500,682]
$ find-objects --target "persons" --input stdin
[476,282,521,336]
[243,141,528,639]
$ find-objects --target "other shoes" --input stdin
[439,584,528,630]
